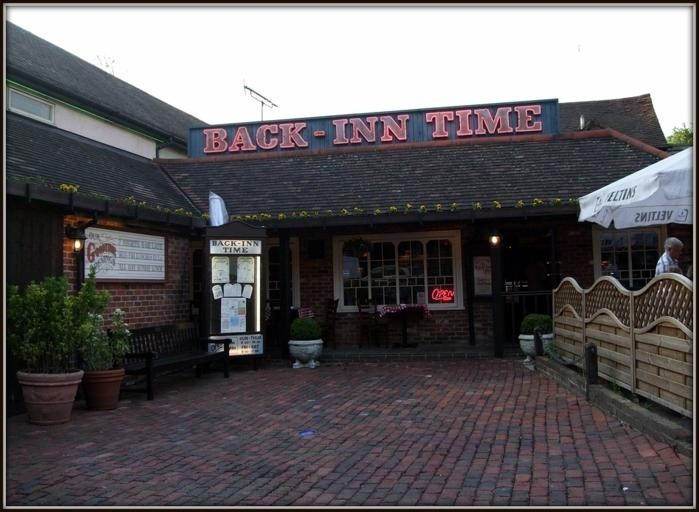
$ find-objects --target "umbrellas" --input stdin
[577,147,693,230]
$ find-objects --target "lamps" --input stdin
[65,224,87,254]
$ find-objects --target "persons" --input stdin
[655,237,684,275]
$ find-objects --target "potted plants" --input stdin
[518,313,554,363]
[287,316,324,369]
[80,308,134,411]
[6,264,109,426]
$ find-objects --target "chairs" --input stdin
[319,298,339,338]
[357,296,388,349]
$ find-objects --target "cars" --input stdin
[343,256,417,297]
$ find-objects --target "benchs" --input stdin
[107,317,232,400]
